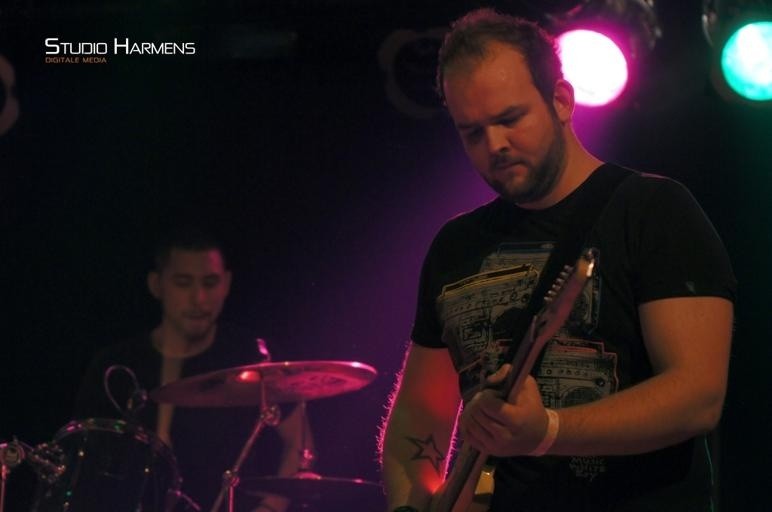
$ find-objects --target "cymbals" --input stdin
[145,360,379,407]
[233,472,382,501]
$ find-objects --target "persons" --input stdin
[73,233,306,512]
[383,8,738,512]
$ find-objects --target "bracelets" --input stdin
[393,506,417,512]
[259,503,276,512]
[528,408,559,456]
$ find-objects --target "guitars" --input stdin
[428,243,596,511]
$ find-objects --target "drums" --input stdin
[24,414,185,512]
